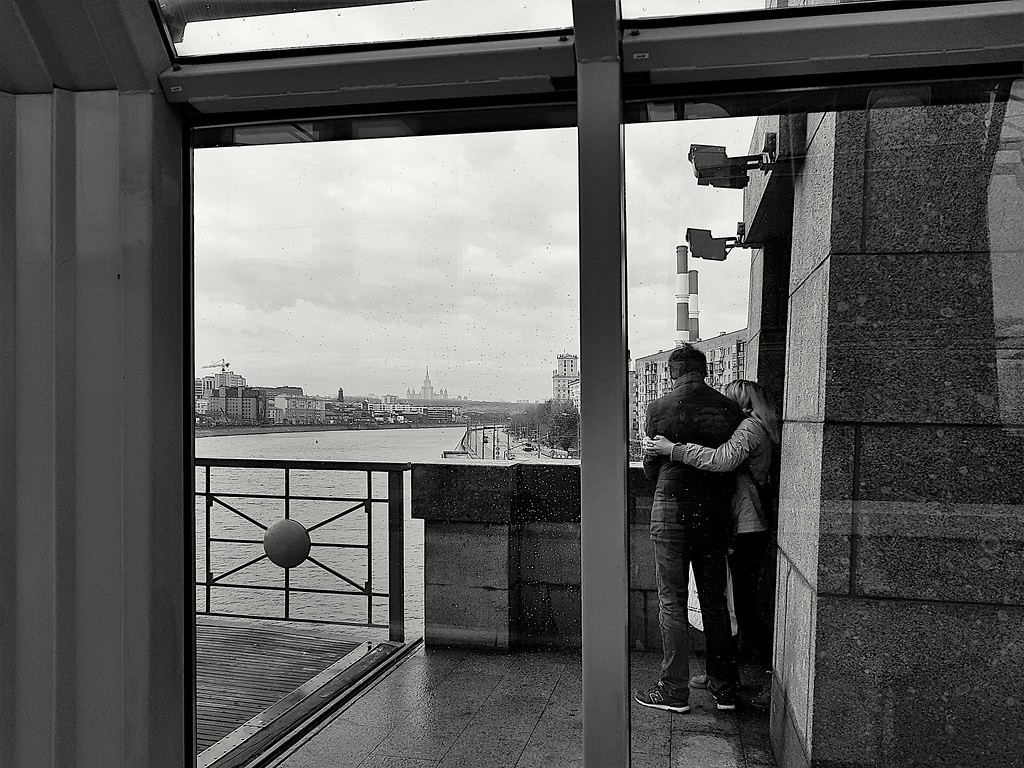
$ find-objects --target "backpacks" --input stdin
[744,421,781,530]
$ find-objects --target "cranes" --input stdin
[200,359,233,372]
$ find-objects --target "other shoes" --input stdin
[690,675,708,689]
[751,683,771,707]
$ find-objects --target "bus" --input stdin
[522,442,532,452]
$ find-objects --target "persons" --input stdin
[645,379,780,709]
[634,344,742,713]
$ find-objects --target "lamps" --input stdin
[687,131,777,189]
[685,222,745,261]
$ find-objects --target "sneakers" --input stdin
[708,681,735,709]
[634,681,690,712]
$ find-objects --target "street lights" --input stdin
[538,413,568,459]
